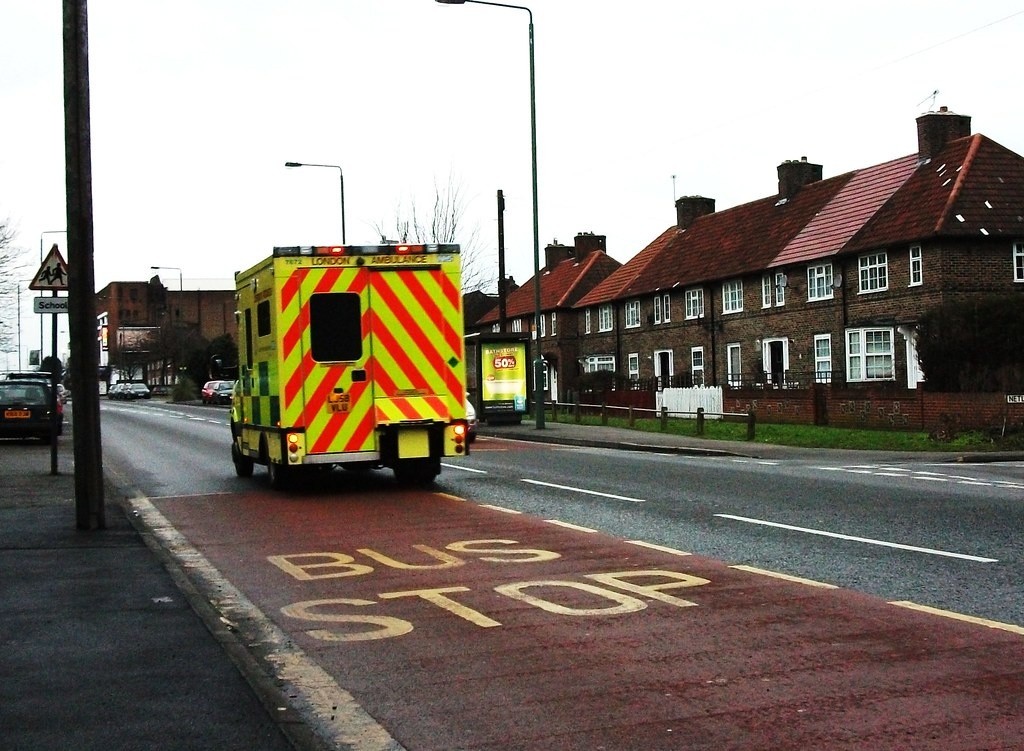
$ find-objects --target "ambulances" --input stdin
[208,231,469,494]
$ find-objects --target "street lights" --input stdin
[151,266,183,332]
[286,162,345,244]
[437,0,546,431]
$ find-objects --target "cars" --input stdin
[0,378,58,445]
[108,382,131,400]
[212,381,236,405]
[201,380,224,405]
[127,383,151,400]
[0,371,71,436]
